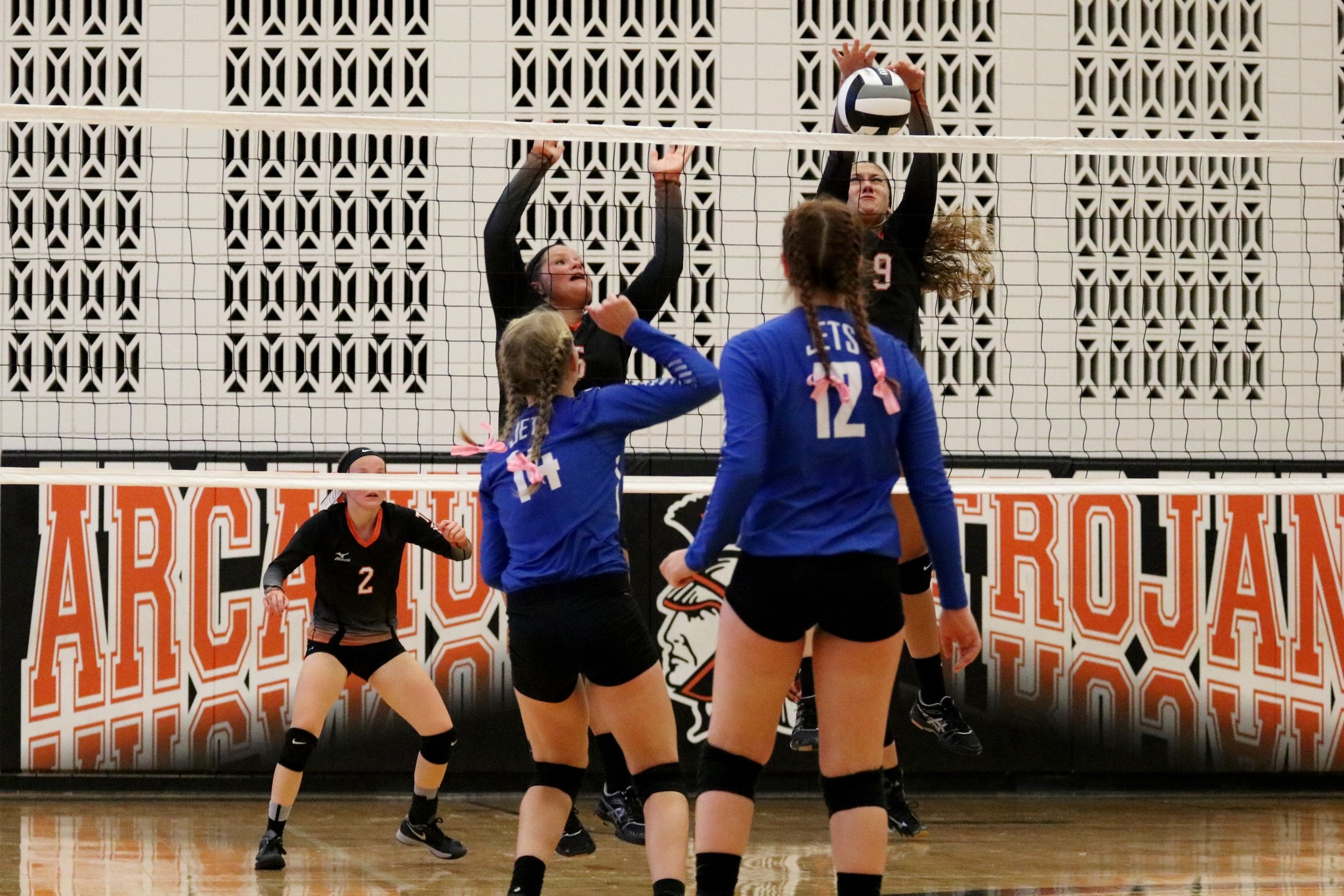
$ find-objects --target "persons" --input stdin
[483,120,695,857]
[253,447,472,869]
[660,39,982,896]
[481,296,723,896]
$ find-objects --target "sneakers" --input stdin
[255,830,287,870]
[790,693,819,751]
[909,690,983,755]
[555,807,596,857]
[884,768,922,838]
[595,781,647,845]
[396,813,467,860]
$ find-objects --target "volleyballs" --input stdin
[838,68,911,137]
